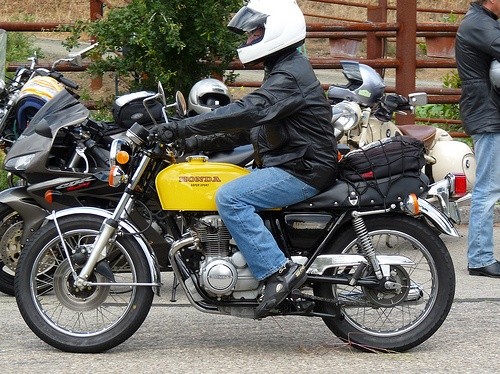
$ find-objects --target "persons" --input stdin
[454,0,500,277]
[149,0,339,319]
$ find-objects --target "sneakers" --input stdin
[256,262,308,318]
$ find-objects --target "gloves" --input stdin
[149,119,179,142]
[175,135,199,158]
[376,92,408,122]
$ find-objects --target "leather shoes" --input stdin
[469,261,500,278]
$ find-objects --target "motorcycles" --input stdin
[0,87,350,302]
[328,85,475,250]
[14,81,467,354]
[0,41,100,153]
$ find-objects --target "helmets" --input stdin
[188,79,232,116]
[489,59,500,94]
[15,76,64,133]
[227,0,307,65]
[328,60,386,106]
